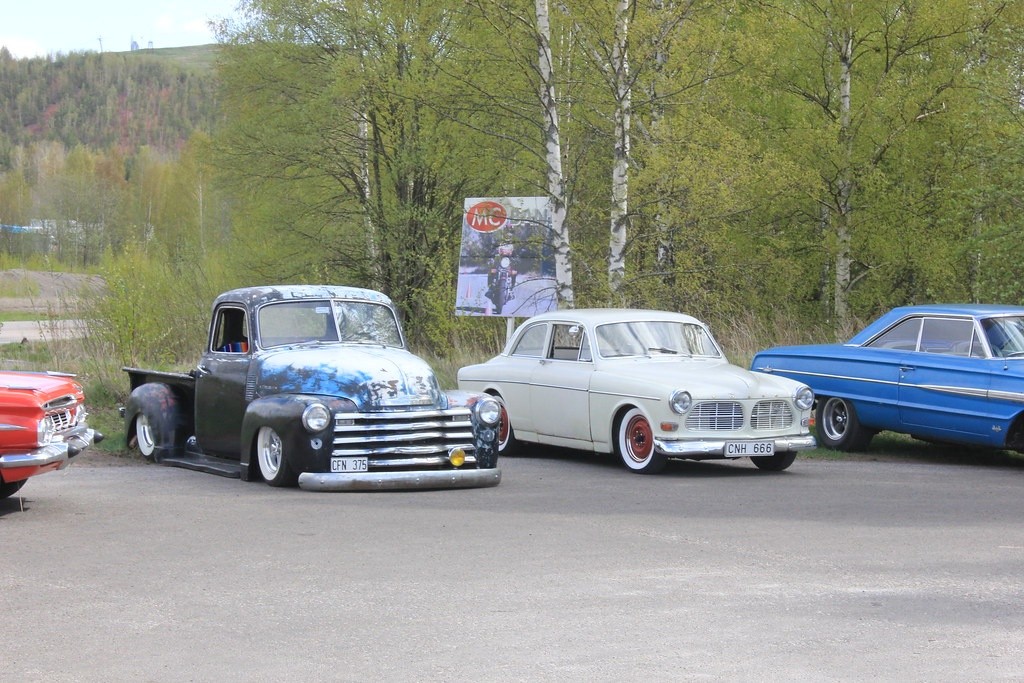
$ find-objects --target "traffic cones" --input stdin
[466,282,472,299]
[483,299,494,315]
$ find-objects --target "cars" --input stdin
[0,370,105,497]
[457,308,818,476]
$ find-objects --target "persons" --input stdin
[487,233,519,288]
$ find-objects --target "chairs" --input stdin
[953,340,1003,358]
[882,342,925,351]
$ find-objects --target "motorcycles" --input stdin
[484,243,519,313]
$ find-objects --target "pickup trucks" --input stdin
[119,283,502,491]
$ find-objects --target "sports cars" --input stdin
[751,304,1024,452]
